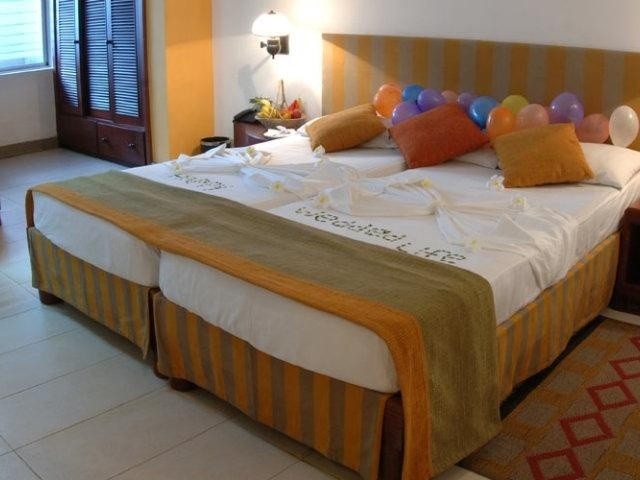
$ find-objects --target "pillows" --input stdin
[490,123,596,187]
[580,143,639,191]
[361,120,398,149]
[387,105,490,169]
[454,143,498,170]
[297,104,388,156]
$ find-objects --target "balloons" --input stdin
[372,83,584,147]
[578,113,609,143]
[609,105,639,149]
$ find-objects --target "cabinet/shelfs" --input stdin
[52,0,216,170]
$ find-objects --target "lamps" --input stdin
[252,8,290,59]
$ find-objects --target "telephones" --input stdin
[234,109,259,123]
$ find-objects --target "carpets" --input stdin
[455,312,639,477]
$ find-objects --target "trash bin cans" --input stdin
[200,136,231,154]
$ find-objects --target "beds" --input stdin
[26,30,639,478]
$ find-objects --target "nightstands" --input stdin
[610,205,640,319]
[232,117,275,149]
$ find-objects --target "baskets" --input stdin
[254,79,307,130]
